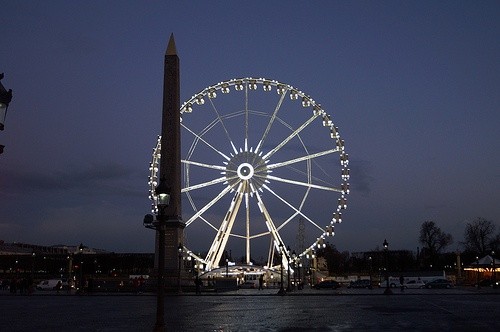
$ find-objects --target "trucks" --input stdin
[36,280,63,290]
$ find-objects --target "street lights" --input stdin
[491,249,497,289]
[368,255,373,289]
[176,244,184,297]
[291,249,303,290]
[476,255,480,289]
[383,240,392,295]
[277,242,286,295]
[286,245,292,292]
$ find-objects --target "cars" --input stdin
[312,280,343,289]
[404,280,426,289]
[347,280,371,288]
[378,279,400,288]
[426,279,455,289]
[471,279,500,288]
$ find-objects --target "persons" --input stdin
[132,279,138,294]
[194,277,201,294]
[258,274,264,290]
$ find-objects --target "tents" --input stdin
[464,255,500,288]
